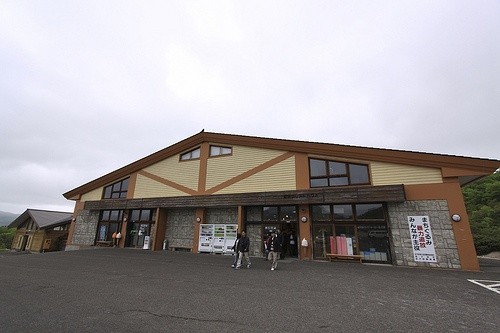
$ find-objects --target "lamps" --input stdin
[301,216,307,223]
[451,214,461,222]
[72,217,76,222]
[124,217,127,222]
[196,217,201,223]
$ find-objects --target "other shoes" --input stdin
[247,265,252,268]
[235,267,241,270]
[231,264,236,268]
[271,268,274,271]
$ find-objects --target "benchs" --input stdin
[326,253,364,264]
[169,246,193,252]
[96,241,112,247]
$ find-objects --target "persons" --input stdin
[231,233,243,268]
[266,232,282,271]
[277,228,297,259]
[99,224,106,241]
[235,230,252,269]
[141,227,147,246]
